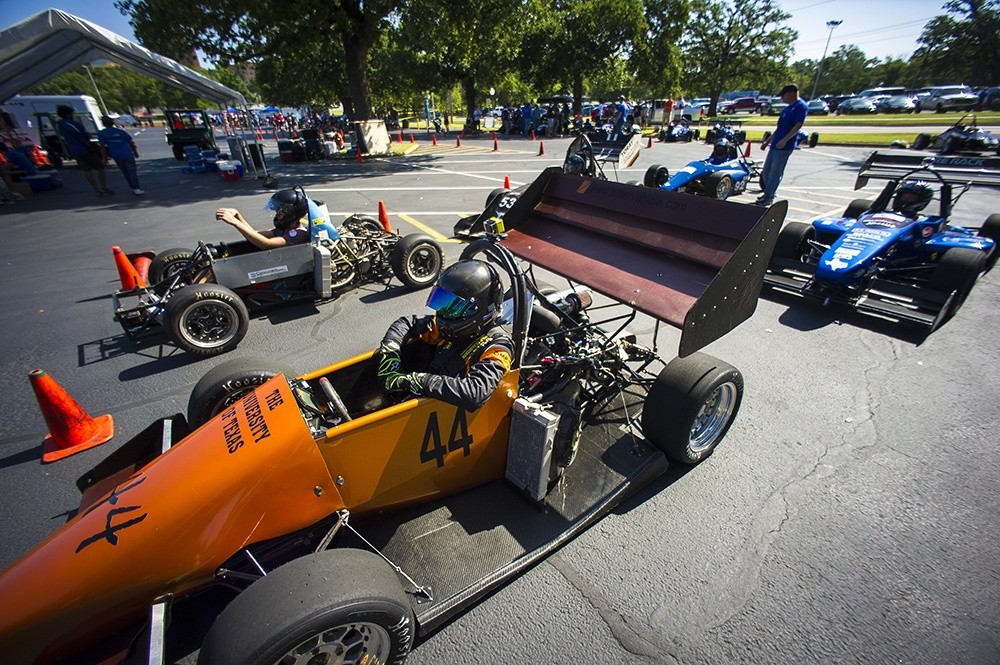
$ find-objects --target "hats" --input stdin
[776,84,798,97]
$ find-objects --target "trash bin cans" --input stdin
[244,143,266,168]
[227,135,249,169]
[301,128,325,160]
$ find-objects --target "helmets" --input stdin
[564,154,584,175]
[270,189,308,232]
[713,138,729,158]
[436,259,504,342]
[893,181,933,215]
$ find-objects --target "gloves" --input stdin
[384,372,422,397]
[375,348,400,383]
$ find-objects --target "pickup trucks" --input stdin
[719,96,769,115]
[163,106,221,162]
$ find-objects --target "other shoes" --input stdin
[757,194,765,200]
[133,189,145,194]
[755,197,773,205]
[102,189,115,195]
[94,189,102,196]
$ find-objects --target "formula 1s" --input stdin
[561,119,1000,337]
[0,159,790,664]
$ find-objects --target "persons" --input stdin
[890,181,935,220]
[97,115,145,195]
[757,84,808,206]
[139,104,351,132]
[381,93,686,138]
[376,259,516,412]
[56,105,114,197]
[217,190,310,249]
[562,154,586,176]
[710,138,731,163]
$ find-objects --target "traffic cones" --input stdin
[491,130,497,139]
[504,176,510,189]
[647,135,652,148]
[492,138,500,152]
[219,123,344,143]
[377,200,395,234]
[355,148,365,163]
[389,132,462,149]
[27,366,116,465]
[113,245,147,291]
[124,250,157,285]
[537,141,545,157]
[530,129,537,141]
[742,140,751,158]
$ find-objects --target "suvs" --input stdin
[767,96,790,116]
[912,85,979,114]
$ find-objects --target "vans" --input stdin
[1,94,107,146]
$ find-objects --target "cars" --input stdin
[111,183,447,360]
[537,94,732,122]
[805,99,831,116]
[758,86,1000,114]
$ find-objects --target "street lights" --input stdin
[809,19,844,99]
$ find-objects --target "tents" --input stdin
[0,7,276,185]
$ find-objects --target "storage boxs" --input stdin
[181,146,244,182]
